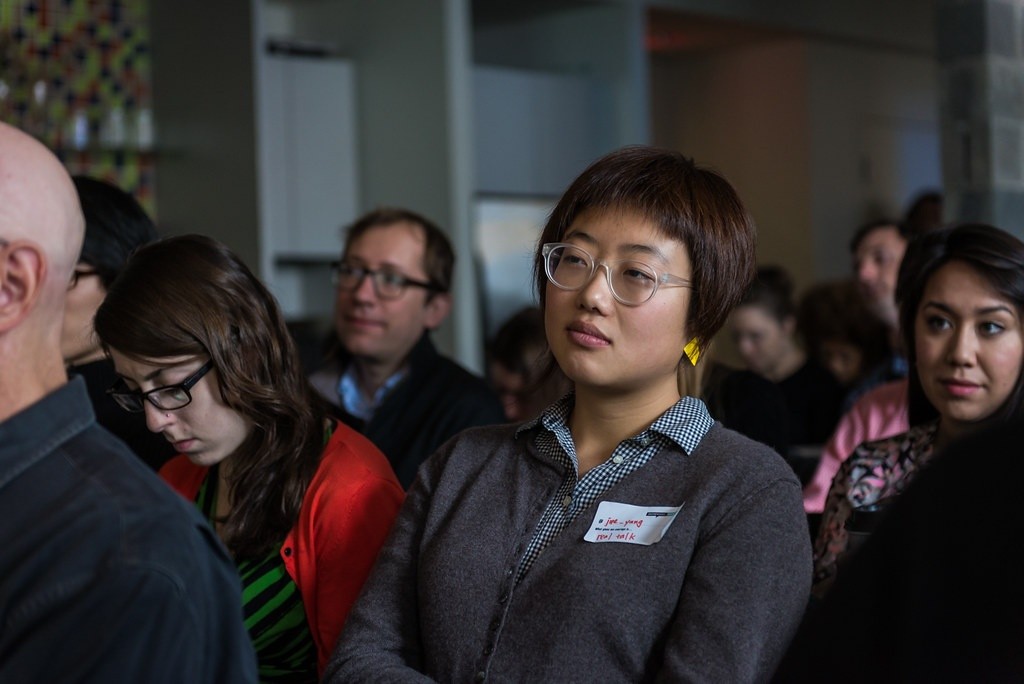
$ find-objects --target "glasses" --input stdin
[67,270,99,290]
[542,244,695,307]
[328,256,438,300]
[106,359,216,414]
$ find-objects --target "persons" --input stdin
[62,148,1024,684]
[0,121,255,684]
